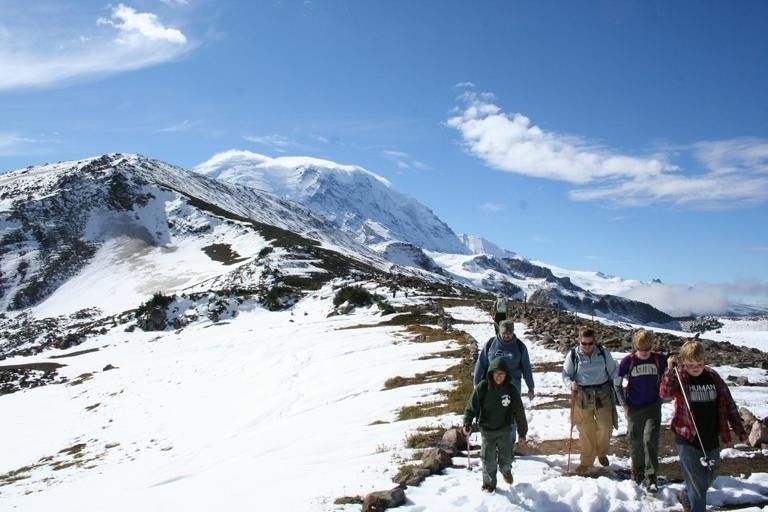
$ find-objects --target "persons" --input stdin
[613,329,670,493]
[658,339,750,512]
[562,325,617,473]
[460,355,529,493]
[471,320,536,448]
[490,293,508,334]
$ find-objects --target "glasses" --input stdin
[635,345,650,351]
[579,338,593,345]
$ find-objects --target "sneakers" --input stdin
[599,457,608,466]
[503,473,513,483]
[576,465,587,474]
[646,474,657,492]
[631,471,644,480]
[681,490,691,512]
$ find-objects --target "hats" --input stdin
[499,320,513,331]
